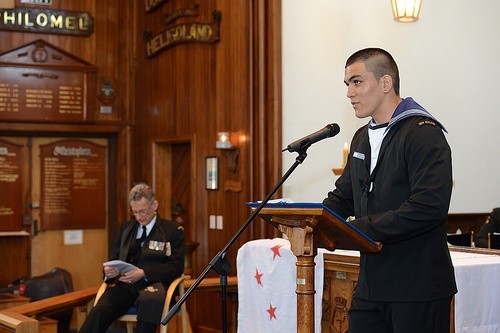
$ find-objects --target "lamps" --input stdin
[215,131,232,149]
[391,0,422,23]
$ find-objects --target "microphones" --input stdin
[281,123,340,152]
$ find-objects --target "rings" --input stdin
[129,279,132,283]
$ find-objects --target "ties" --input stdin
[136,225,146,249]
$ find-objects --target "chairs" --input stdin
[93,226,192,333]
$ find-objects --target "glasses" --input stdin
[130,200,154,215]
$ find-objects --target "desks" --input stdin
[236,238,500,333]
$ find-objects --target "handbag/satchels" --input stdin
[19,268,74,316]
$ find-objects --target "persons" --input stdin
[79,184,184,333]
[318,48,458,333]
